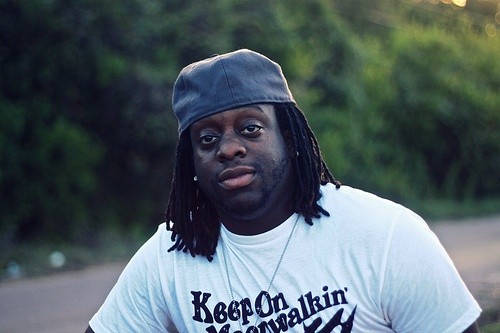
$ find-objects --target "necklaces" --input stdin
[220,210,300,333]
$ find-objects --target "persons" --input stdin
[84,49,484,333]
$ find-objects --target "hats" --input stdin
[172,49,296,136]
